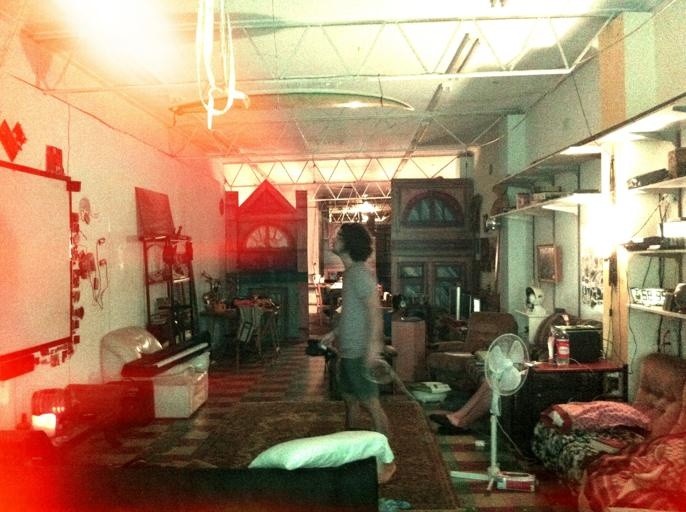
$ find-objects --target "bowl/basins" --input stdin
[621,242,652,251]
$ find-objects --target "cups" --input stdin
[643,236,663,250]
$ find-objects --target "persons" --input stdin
[377,282,392,302]
[319,221,397,486]
[430,313,575,433]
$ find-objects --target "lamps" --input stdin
[169,0,414,115]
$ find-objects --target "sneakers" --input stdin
[429,413,471,434]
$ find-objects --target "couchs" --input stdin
[558,354,686,512]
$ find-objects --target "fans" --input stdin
[450,334,539,493]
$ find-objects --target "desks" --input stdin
[527,360,628,461]
[200,306,280,372]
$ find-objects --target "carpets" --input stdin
[117,399,462,512]
[403,381,577,512]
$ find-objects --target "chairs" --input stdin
[425,311,518,399]
[100,325,210,420]
[465,313,582,386]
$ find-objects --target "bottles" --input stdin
[555,326,570,367]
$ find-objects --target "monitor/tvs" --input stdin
[473,297,482,312]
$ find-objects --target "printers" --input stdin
[550,323,603,363]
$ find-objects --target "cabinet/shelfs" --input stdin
[139,234,199,346]
[490,92,686,406]
[475,232,499,289]
[390,178,475,322]
